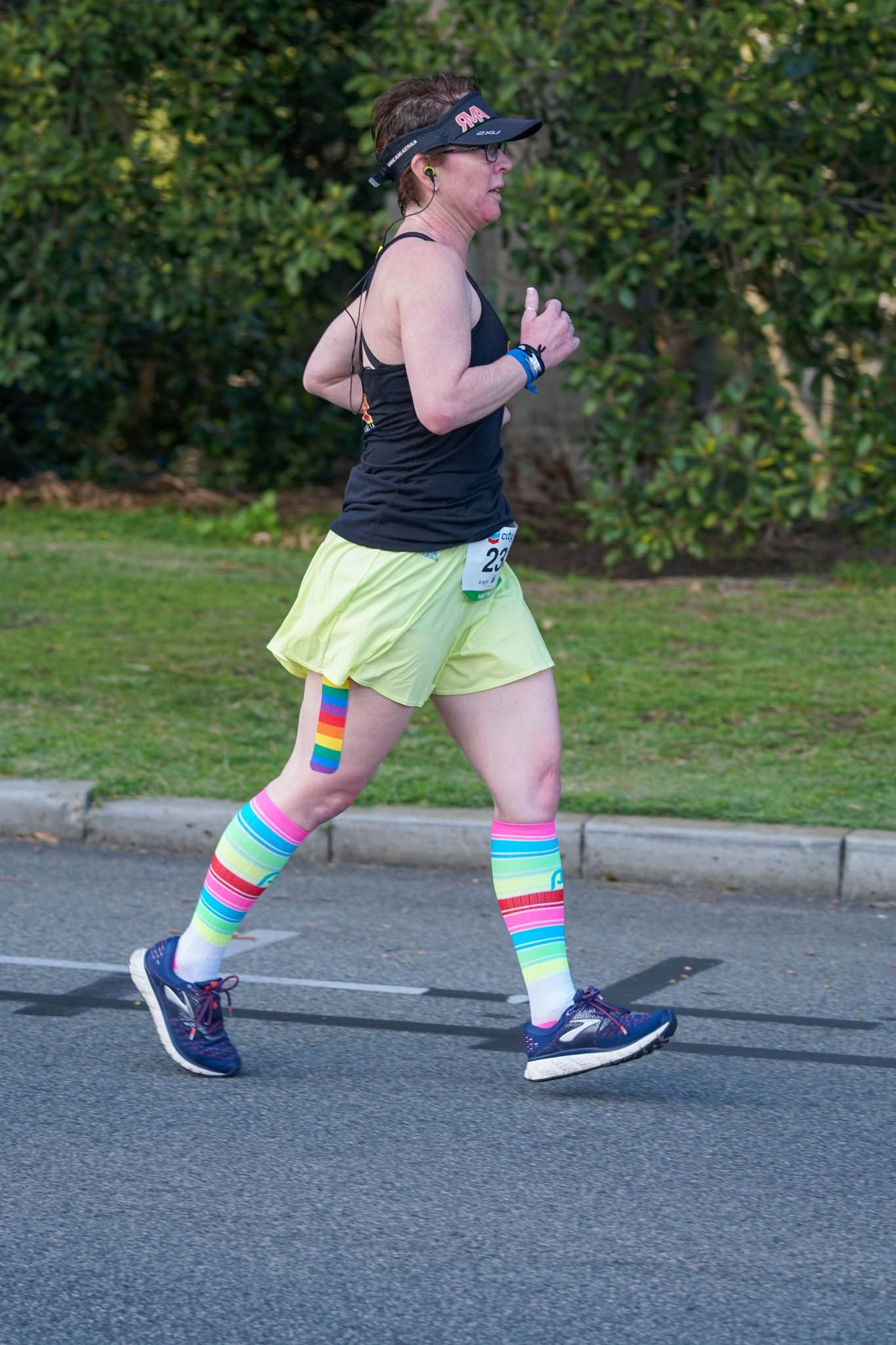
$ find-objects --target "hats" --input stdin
[368,92,545,188]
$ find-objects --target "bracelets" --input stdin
[507,342,545,394]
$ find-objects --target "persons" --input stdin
[125,72,678,1087]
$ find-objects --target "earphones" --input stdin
[425,166,433,177]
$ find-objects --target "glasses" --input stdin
[432,141,509,162]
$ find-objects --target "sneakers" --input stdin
[521,986,677,1082]
[129,935,241,1076]
